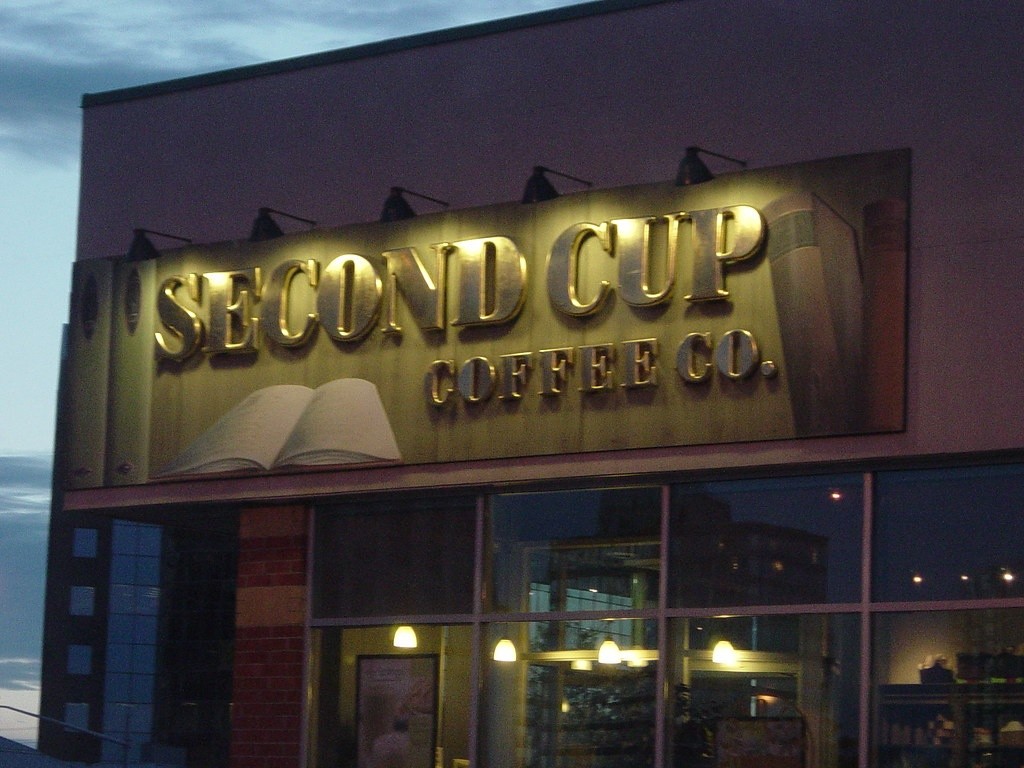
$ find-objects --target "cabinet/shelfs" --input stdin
[869,684,1024,768]
[525,663,654,768]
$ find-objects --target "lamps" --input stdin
[521,166,594,206]
[247,209,318,242]
[572,544,650,672]
[490,519,521,665]
[673,147,745,189]
[122,229,191,263]
[380,185,450,224]
